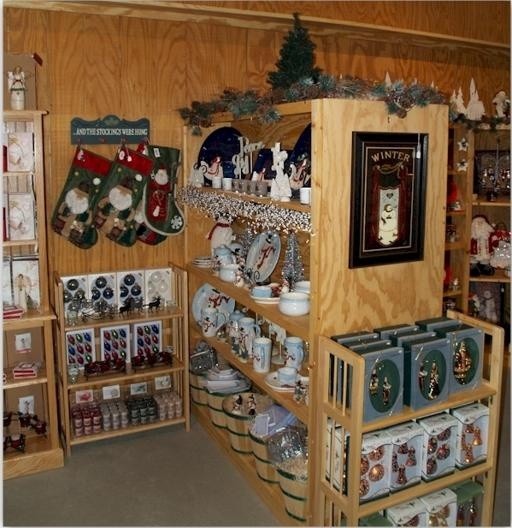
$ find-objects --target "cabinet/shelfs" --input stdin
[470,120,512,368]
[2,109,66,481]
[444,121,477,317]
[311,311,504,527]
[51,261,190,458]
[181,99,451,527]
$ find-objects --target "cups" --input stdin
[190,282,310,406]
[213,177,311,205]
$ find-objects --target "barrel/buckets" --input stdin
[205,379,247,426]
[278,462,309,519]
[221,392,271,452]
[248,426,281,481]
[189,371,208,404]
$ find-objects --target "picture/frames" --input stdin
[348,131,429,269]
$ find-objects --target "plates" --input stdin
[192,230,311,304]
[197,127,244,186]
[287,123,310,192]
